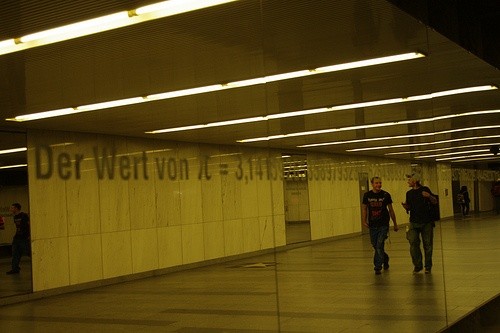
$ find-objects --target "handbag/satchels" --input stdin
[421,186,440,221]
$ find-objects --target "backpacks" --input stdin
[456,191,467,204]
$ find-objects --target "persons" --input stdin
[361,177,399,273]
[457,185,471,217]
[401,173,438,274]
[6,203,30,275]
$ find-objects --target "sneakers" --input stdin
[425,266,431,273]
[413,266,423,272]
[374,264,382,271]
[383,255,389,270]
